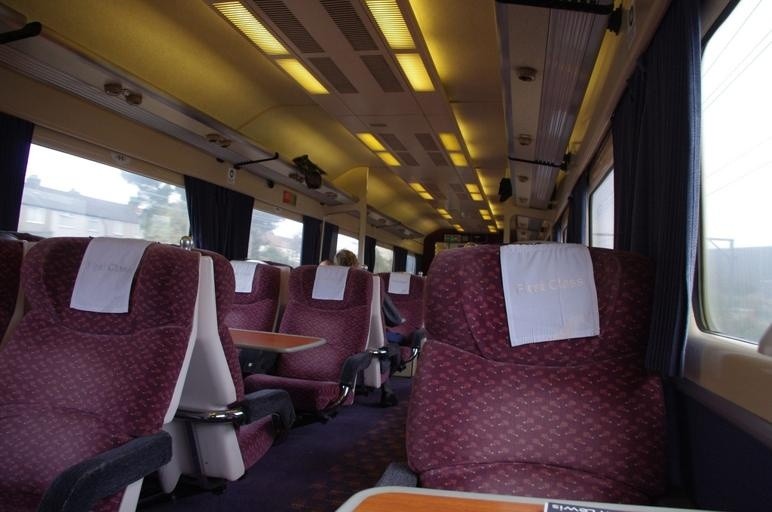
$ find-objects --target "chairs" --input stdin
[336,230,694,512]
[0,231,423,512]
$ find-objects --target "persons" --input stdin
[317,249,401,329]
